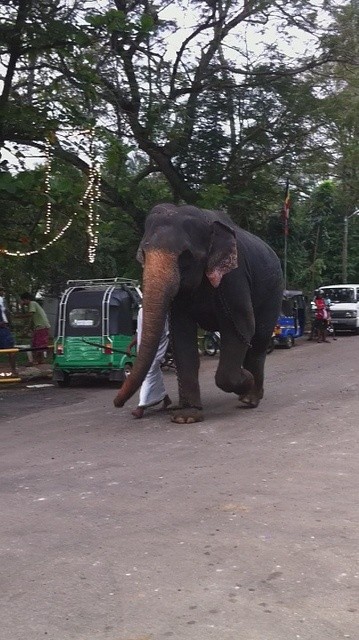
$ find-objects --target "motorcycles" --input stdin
[204,329,221,356]
[311,301,333,343]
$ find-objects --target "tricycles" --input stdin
[272,290,308,348]
[54,277,143,388]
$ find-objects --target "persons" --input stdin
[0,292,17,376]
[308,292,338,341]
[127,303,172,419]
[16,292,51,367]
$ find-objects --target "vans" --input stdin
[310,284,359,334]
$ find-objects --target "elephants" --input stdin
[113,202,284,424]
